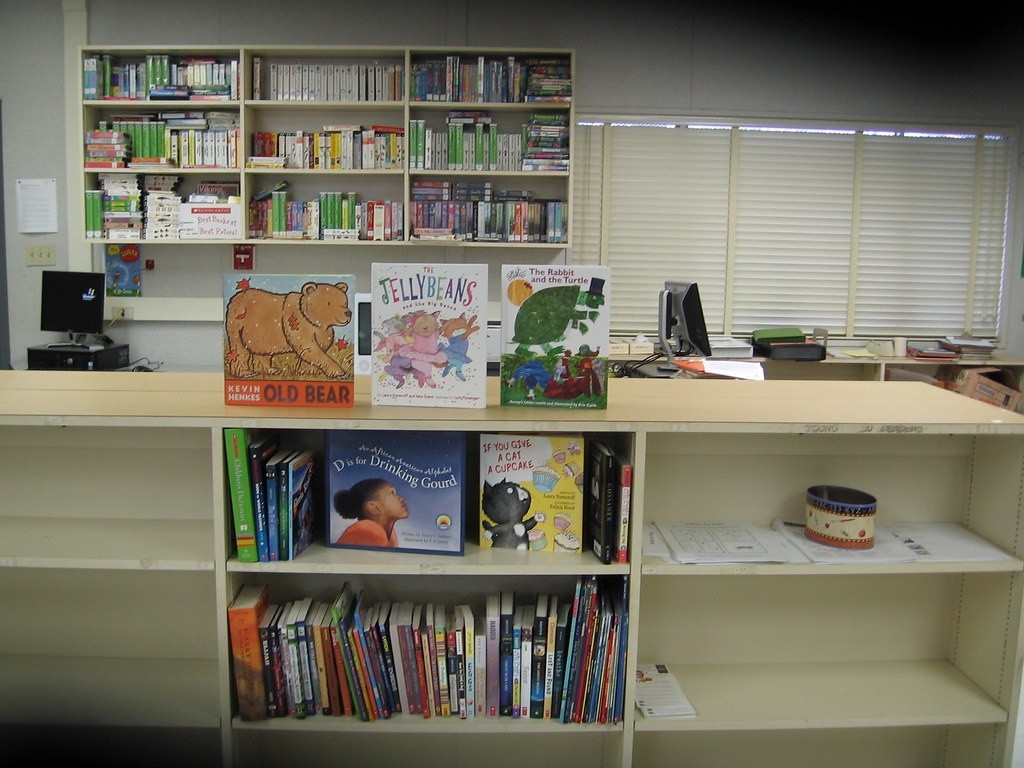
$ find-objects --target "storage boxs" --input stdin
[953,367,1023,412]
[709,335,753,358]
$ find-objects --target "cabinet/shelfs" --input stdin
[77,44,576,248]
[0,368,1024,768]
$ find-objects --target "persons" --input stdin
[335,479,408,548]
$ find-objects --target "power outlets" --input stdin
[112,306,134,320]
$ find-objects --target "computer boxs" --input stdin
[28,344,130,372]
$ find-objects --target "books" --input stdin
[906,337,995,360]
[635,657,697,719]
[222,274,355,408]
[409,57,570,244]
[500,265,612,409]
[245,57,405,242]
[371,263,489,409]
[225,427,634,565]
[83,54,240,298]
[228,575,630,726]
[707,328,826,361]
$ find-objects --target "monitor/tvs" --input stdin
[655,281,712,373]
[40,270,106,351]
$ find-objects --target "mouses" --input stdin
[132,365,154,373]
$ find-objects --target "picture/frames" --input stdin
[324,429,466,556]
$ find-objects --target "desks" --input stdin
[609,353,1024,412]
